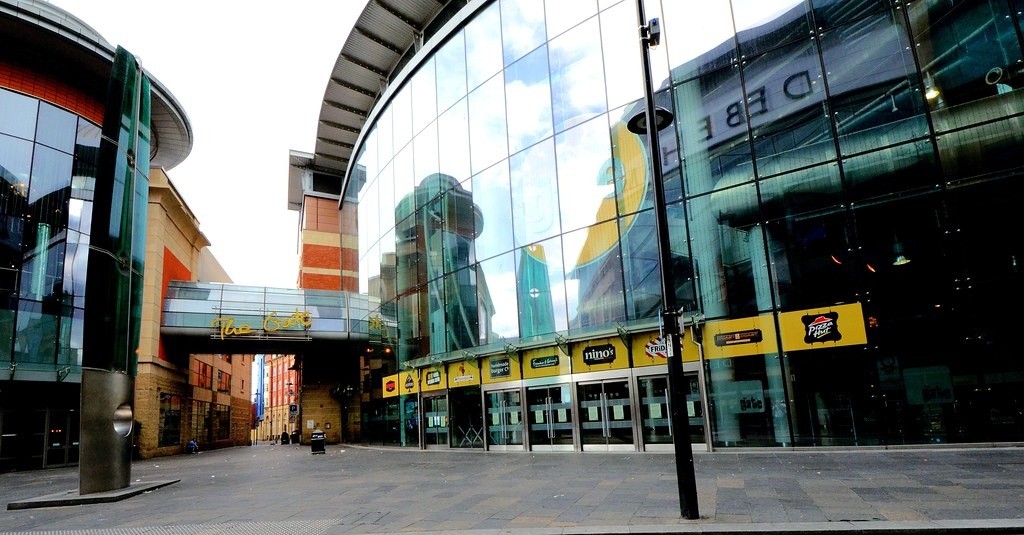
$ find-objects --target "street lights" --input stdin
[626,103,700,519]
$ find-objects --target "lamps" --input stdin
[924,72,939,100]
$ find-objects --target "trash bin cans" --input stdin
[310,438,325,455]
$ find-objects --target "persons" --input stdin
[316,423,320,429]
[187,438,199,454]
